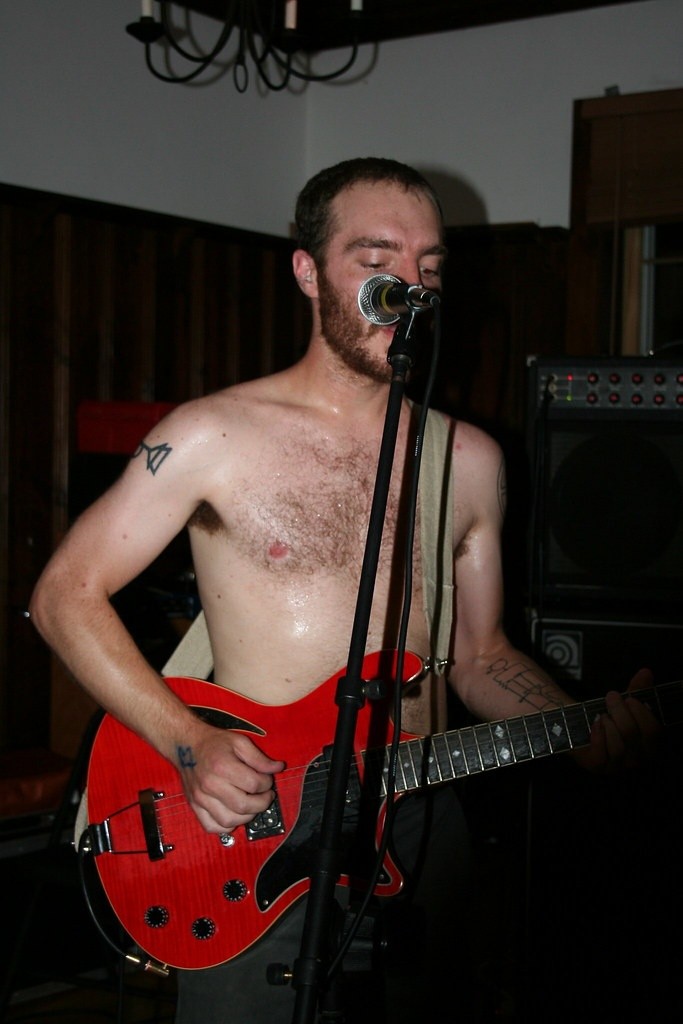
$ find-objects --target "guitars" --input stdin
[82,641,681,978]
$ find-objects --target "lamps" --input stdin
[125,0,364,94]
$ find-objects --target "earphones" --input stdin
[302,275,311,282]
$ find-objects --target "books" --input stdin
[621,222,683,359]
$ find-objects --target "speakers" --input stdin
[516,616,682,1023]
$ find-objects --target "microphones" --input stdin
[357,274,441,326]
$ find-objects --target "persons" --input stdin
[28,157,658,1024]
